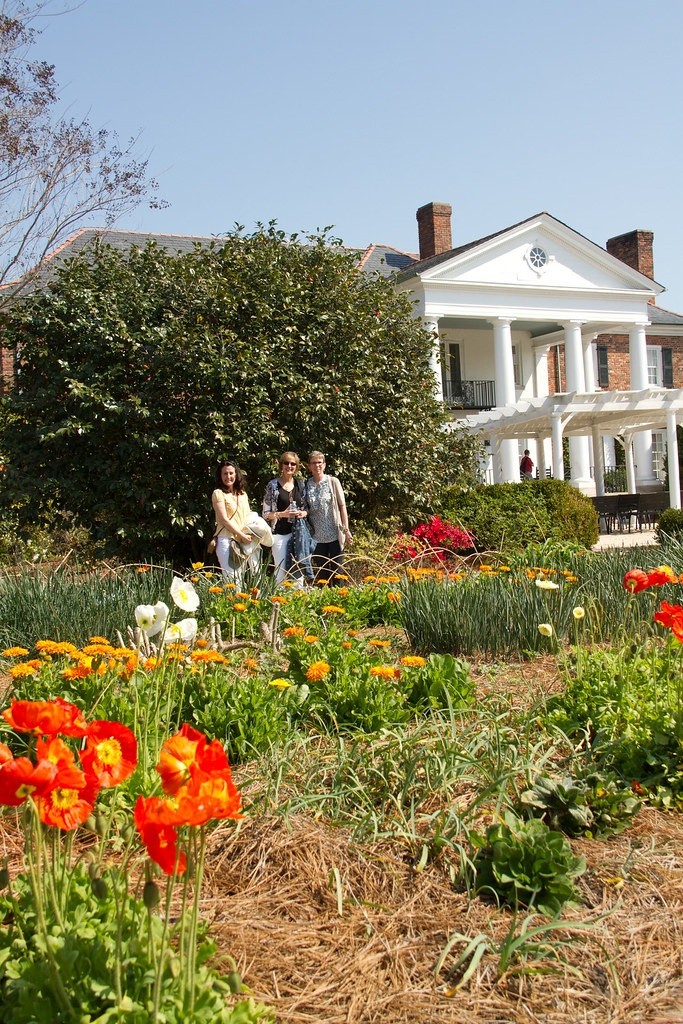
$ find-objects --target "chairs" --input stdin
[536,468,552,479]
[591,492,665,534]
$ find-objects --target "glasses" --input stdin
[282,461,296,466]
[309,462,323,465]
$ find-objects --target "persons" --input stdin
[262,452,317,586]
[305,451,354,589]
[212,461,264,585]
[521,449,534,481]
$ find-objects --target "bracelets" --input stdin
[343,529,349,532]
[274,512,280,520]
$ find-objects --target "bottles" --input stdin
[288,501,297,523]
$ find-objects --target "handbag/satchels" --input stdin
[207,536,218,554]
[520,457,528,475]
